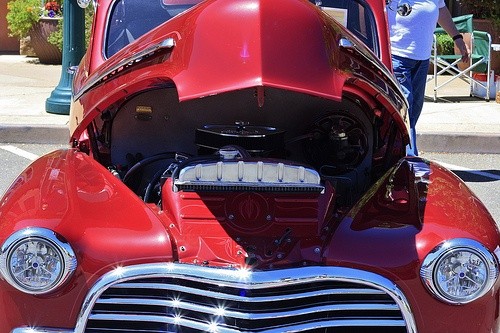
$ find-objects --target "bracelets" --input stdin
[452,34,463,41]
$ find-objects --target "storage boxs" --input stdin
[472,72,497,99]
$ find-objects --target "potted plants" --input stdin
[6,0,95,65]
[454,0,500,77]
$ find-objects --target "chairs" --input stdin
[425,15,492,103]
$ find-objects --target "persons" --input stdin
[385,0,469,157]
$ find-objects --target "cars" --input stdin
[0,0,499,333]
